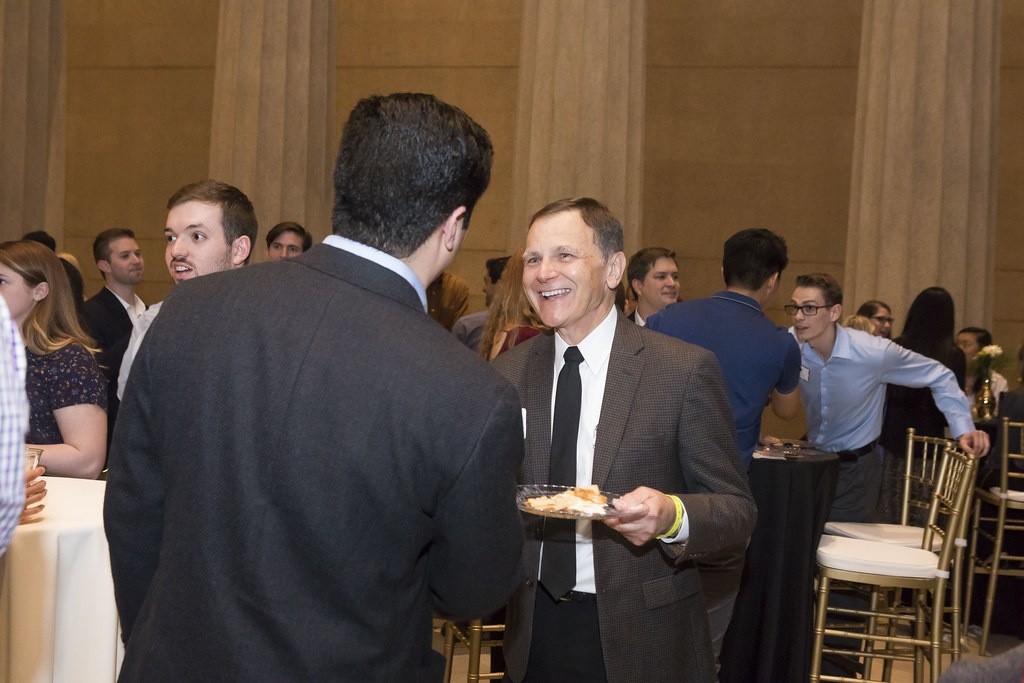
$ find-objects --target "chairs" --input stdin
[807,417,1024,683]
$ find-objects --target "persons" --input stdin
[788,274,1024,683]
[265,222,311,262]
[425,256,541,362]
[626,247,681,326]
[490,198,755,683]
[644,228,802,670]
[87,229,149,431]
[117,178,258,401]
[104,93,529,682]
[0,230,109,564]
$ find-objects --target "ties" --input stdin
[539,346,584,602]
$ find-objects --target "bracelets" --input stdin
[655,496,682,539]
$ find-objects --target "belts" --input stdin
[836,440,878,462]
[538,582,596,606]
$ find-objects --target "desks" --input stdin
[0,475,126,683]
[710,437,838,683]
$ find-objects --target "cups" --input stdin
[26,447,45,511]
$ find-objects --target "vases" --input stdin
[977,377,996,421]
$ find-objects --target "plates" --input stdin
[763,447,826,457]
[759,438,816,449]
[513,483,622,521]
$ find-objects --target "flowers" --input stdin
[966,342,1003,379]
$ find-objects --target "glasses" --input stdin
[784,305,833,316]
[870,316,894,326]
